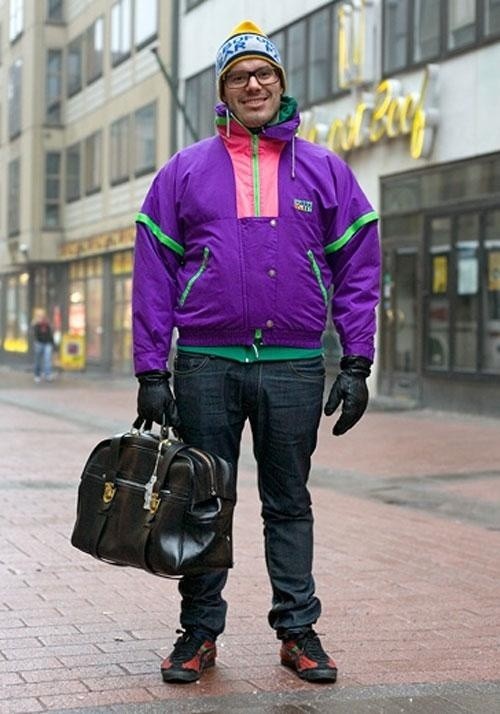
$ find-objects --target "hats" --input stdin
[214,19,287,104]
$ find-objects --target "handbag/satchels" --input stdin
[70,406,238,579]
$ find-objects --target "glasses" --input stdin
[222,65,281,90]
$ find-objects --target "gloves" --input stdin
[134,369,183,428]
[323,354,374,437]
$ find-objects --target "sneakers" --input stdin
[160,633,218,683]
[279,634,338,682]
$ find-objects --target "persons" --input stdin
[32,306,58,382]
[135,20,383,686]
